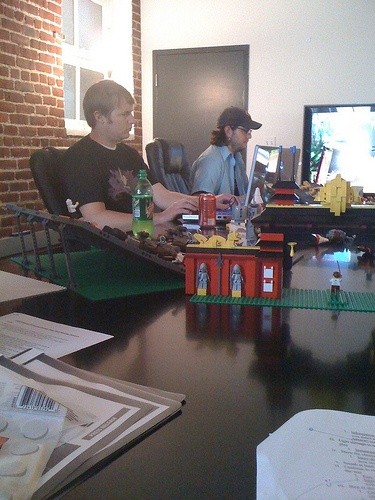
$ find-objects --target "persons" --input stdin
[189,106,262,200]
[56,81,241,251]
[232,264,244,290]
[329,272,343,295]
[198,262,209,289]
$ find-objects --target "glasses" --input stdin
[235,127,252,138]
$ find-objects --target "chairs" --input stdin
[29,148,66,214]
[145,137,191,197]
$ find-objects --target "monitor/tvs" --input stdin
[278,148,300,181]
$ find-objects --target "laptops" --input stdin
[182,146,259,221]
[268,147,333,205]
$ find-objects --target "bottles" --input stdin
[132,169,153,241]
[251,187,264,235]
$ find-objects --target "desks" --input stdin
[0,221,375,500]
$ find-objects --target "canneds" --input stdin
[199,193,216,229]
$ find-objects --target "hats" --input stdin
[216,106,262,130]
